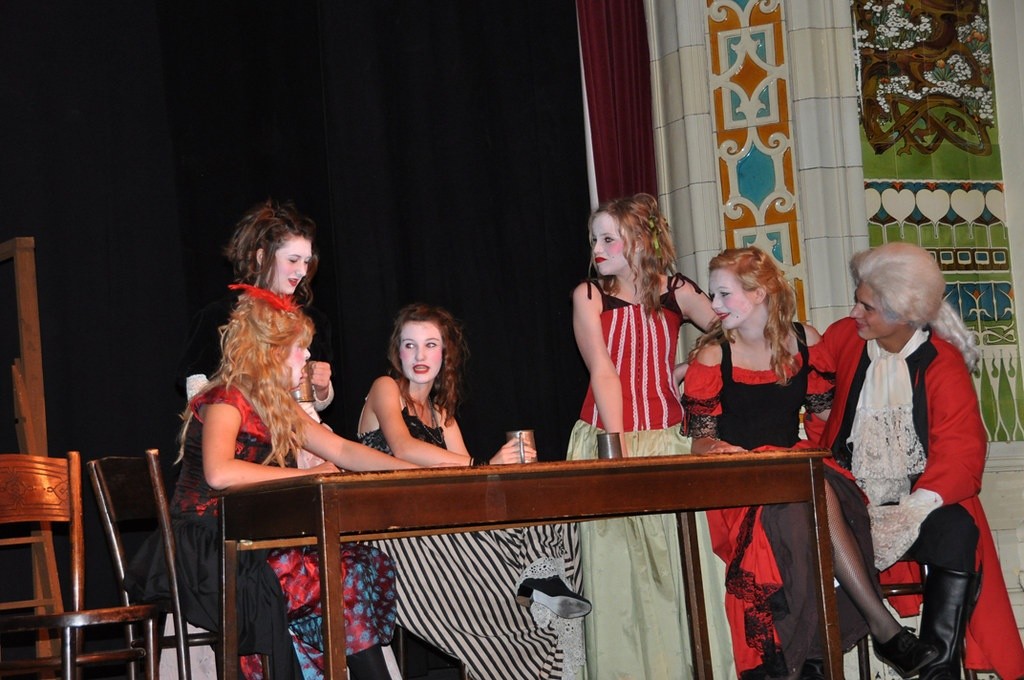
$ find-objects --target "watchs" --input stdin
[680,246,939,680]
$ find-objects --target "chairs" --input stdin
[87,449,271,680]
[858,557,977,680]
[1,450,161,680]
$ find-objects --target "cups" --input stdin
[506,429,537,463]
[289,360,316,402]
[597,432,622,459]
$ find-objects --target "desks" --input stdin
[206,448,844,680]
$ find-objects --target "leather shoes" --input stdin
[873,625,939,678]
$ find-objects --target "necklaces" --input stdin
[407,386,445,445]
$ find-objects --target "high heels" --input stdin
[517,573,592,621]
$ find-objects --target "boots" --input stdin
[740,655,825,680]
[919,566,978,680]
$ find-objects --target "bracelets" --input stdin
[470,457,490,466]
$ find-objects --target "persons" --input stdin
[353,304,592,680]
[120,283,448,680]
[566,194,740,680]
[789,242,1024,680]
[224,199,396,680]
